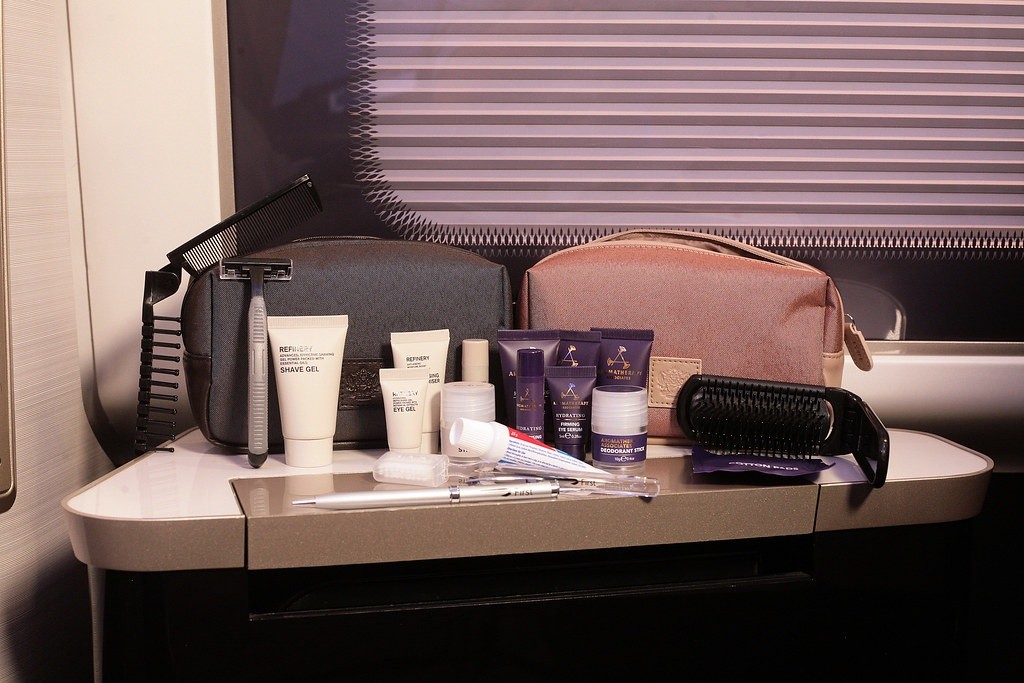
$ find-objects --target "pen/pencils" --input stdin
[291,476,562,510]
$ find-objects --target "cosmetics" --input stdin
[590,386,649,465]
[390,330,451,455]
[463,340,489,379]
[379,369,431,453]
[545,366,594,462]
[498,327,559,434]
[552,329,602,365]
[592,326,655,388]
[442,379,495,466]
[267,313,351,469]
[450,416,611,482]
[516,348,544,441]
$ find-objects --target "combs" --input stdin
[128,173,314,459]
[674,369,891,495]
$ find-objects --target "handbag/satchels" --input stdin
[181,235,508,453]
[518,231,873,446]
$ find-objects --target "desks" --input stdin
[69,425,995,683]
